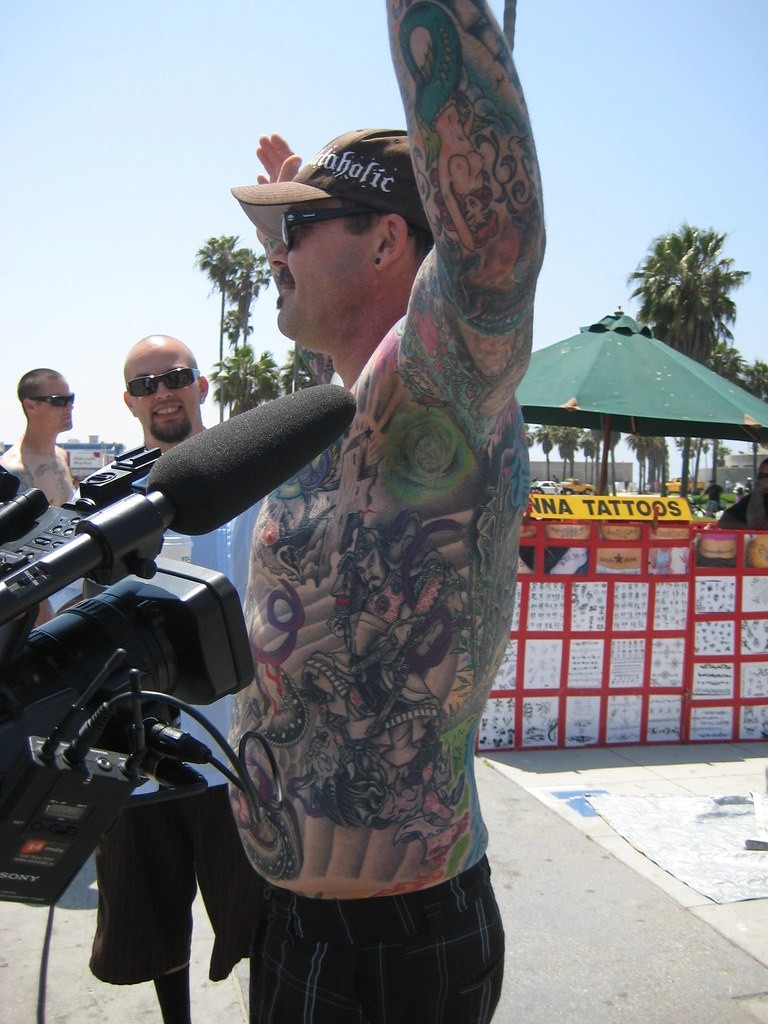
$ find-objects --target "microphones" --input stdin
[0,385,357,625]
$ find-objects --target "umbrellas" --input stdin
[514,306,768,492]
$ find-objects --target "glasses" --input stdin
[126,368,200,397]
[280,205,383,251]
[29,393,75,407]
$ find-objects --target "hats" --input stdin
[231,126,433,238]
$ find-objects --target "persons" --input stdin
[0,368,75,507]
[31,335,263,1024]
[227,0,546,1024]
[525,457,768,530]
